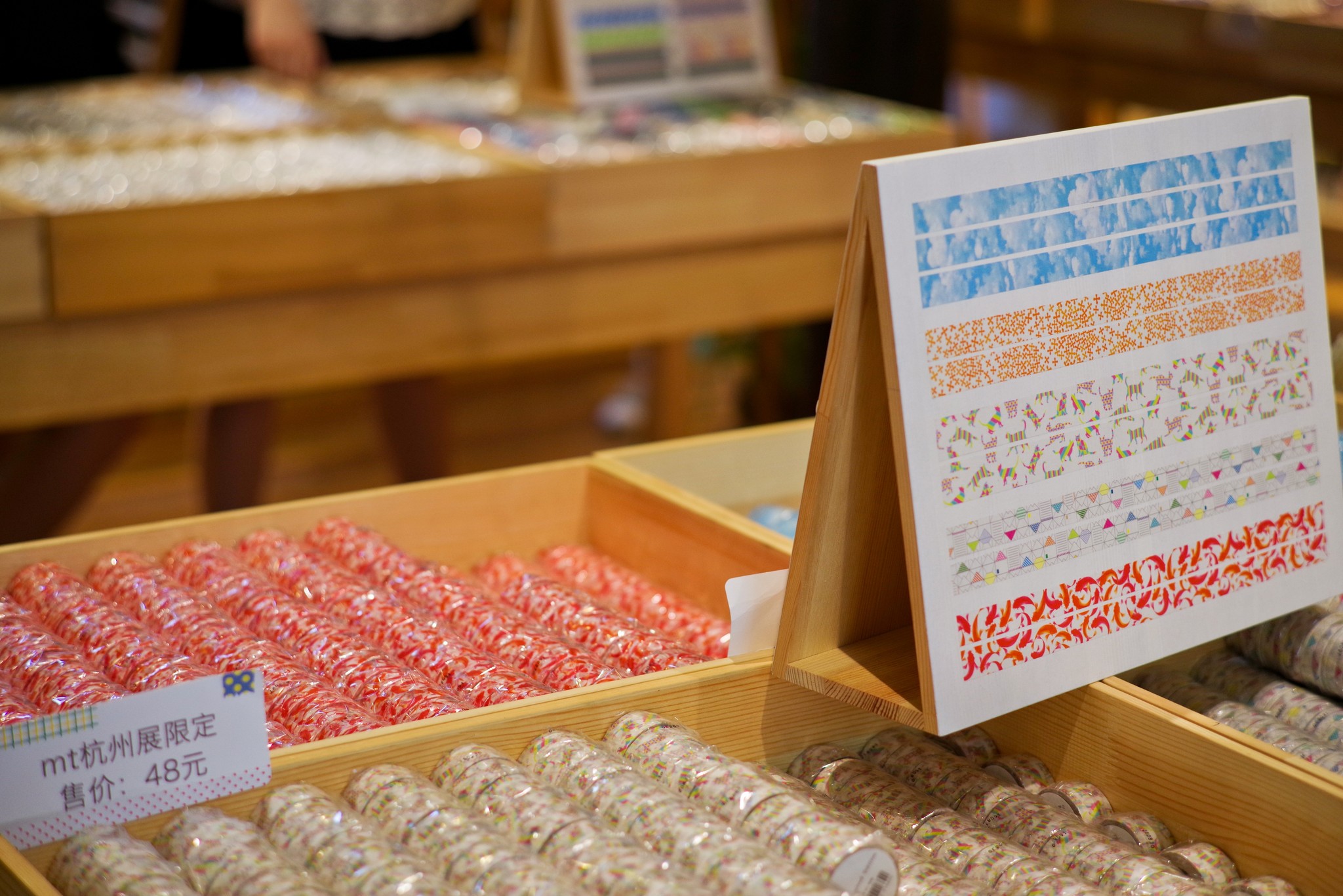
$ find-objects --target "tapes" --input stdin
[0,493,1343,896]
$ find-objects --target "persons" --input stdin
[245,0,486,79]
[792,0,952,113]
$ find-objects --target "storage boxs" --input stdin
[0,59,1343,896]
